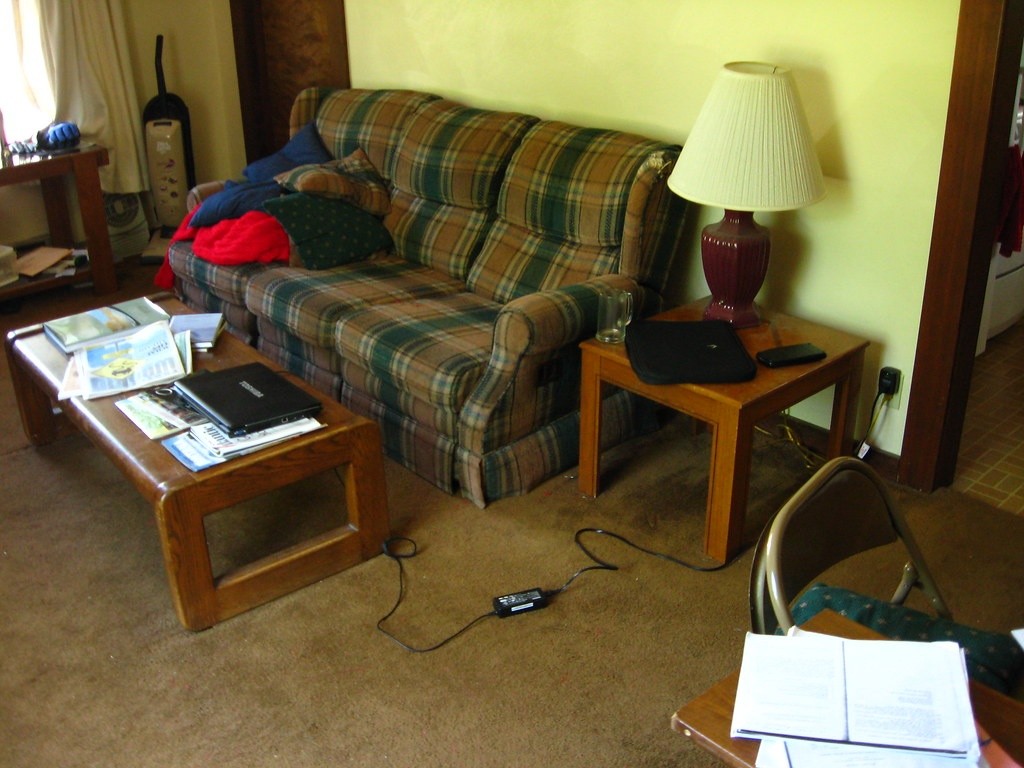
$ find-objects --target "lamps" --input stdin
[666,61,827,330]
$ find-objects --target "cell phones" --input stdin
[756,343,829,368]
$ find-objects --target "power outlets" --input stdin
[882,376,903,409]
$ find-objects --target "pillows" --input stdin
[242,121,335,185]
[259,191,393,271]
[188,179,295,229]
[272,147,394,214]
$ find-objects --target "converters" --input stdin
[493,588,548,618]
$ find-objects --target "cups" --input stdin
[596,288,634,344]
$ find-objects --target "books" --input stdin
[43,297,320,472]
[728,625,981,768]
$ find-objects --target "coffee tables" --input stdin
[7,292,387,629]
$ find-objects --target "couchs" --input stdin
[170,86,688,505]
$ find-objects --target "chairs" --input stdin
[749,457,1024,701]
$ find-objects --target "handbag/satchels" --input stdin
[625,318,758,385]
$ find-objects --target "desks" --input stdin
[672,605,1024,768]
[580,293,868,564]
[0,139,119,302]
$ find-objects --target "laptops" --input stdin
[174,361,323,438]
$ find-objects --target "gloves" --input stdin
[37,121,81,150]
